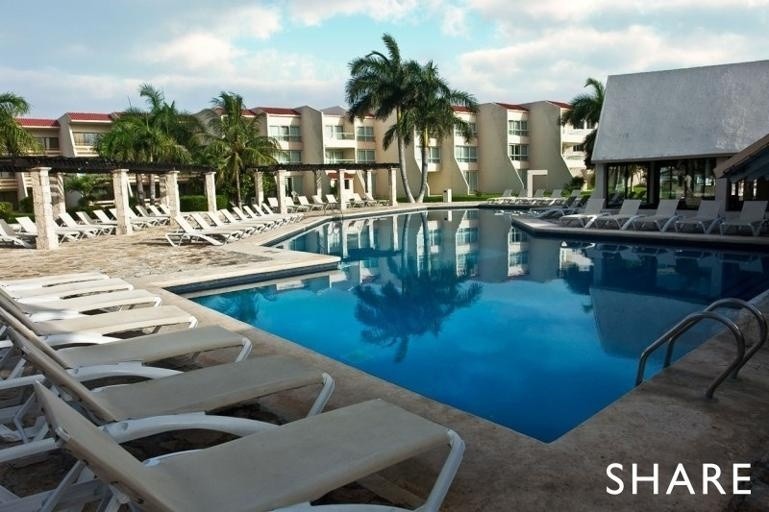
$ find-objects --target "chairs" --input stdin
[486,190,769,237]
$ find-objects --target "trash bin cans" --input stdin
[251,197,256,204]
[442,188,452,203]
